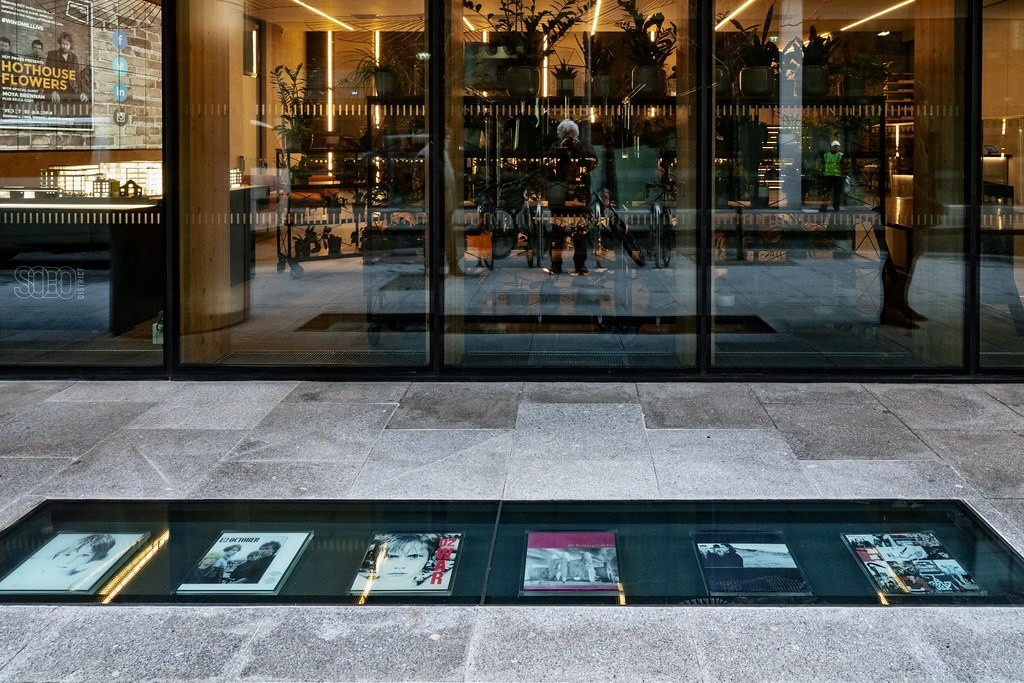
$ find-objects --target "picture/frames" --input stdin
[349,531,466,594]
[518,529,621,596]
[0,528,152,597]
[840,531,988,597]
[691,530,815,597]
[172,527,314,597]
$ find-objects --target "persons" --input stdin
[545,120,598,273]
[871,80,931,330]
[818,141,847,213]
[412,125,466,277]
[847,534,981,592]
[358,532,441,589]
[13,534,116,592]
[699,544,743,568]
[0,32,92,129]
[199,541,280,585]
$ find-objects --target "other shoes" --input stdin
[543,267,562,275]
[568,267,590,276]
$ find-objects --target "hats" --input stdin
[831,140,840,147]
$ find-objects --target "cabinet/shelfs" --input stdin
[882,73,915,120]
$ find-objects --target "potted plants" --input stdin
[614,0,676,96]
[715,2,890,99]
[551,58,579,97]
[462,0,596,102]
[269,63,315,151]
[462,110,681,207]
[714,116,865,261]
[293,225,342,259]
[290,114,427,214]
[339,45,427,98]
[574,32,615,96]
[351,216,675,256]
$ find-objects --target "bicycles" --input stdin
[476,178,678,271]
